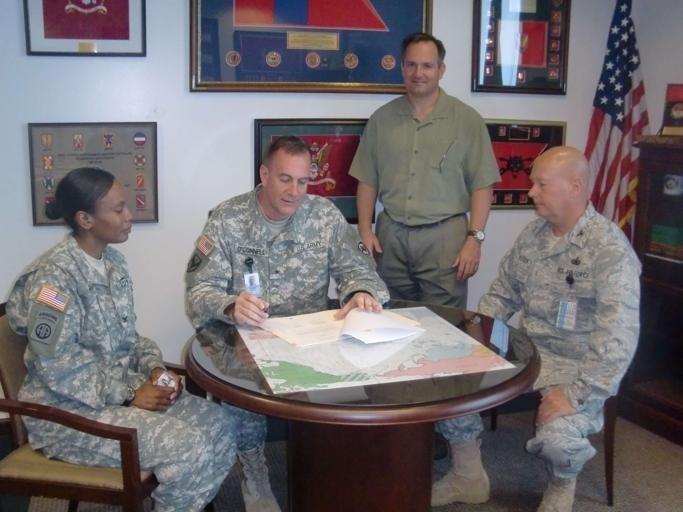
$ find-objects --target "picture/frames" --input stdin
[188,1,433,94]
[28,121,159,227]
[23,0,147,58]
[484,117,568,211]
[471,0,571,97]
[254,118,376,225]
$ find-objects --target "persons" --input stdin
[347,32,503,311]
[184,135,391,512]
[5,168,283,511]
[431,146,644,512]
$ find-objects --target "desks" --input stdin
[185,299,541,511]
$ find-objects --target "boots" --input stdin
[237,442,281,512]
[431,437,491,508]
[537,476,576,512]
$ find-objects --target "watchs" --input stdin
[466,229,485,243]
[122,386,137,406]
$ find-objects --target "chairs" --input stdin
[1,301,220,512]
[481,393,621,506]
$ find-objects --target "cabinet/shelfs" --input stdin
[616,135,683,446]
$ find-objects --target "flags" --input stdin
[585,0,649,246]
